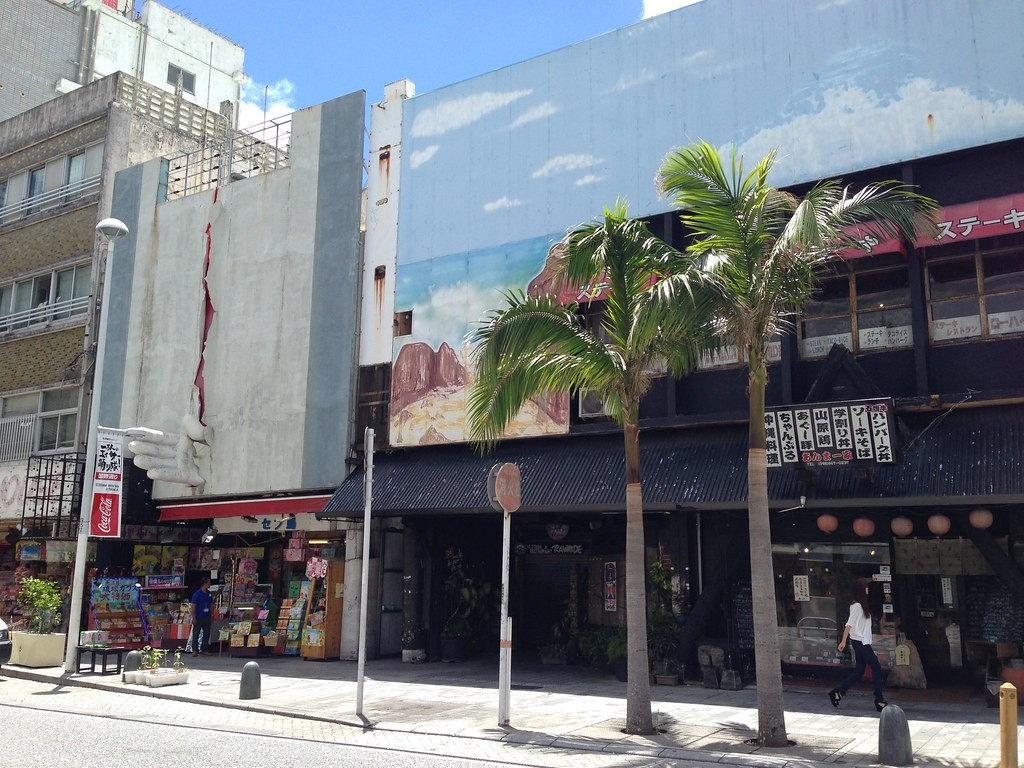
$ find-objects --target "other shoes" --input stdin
[192,650,198,657]
[202,650,213,656]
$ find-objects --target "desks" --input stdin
[965,640,1020,689]
[75,646,125,676]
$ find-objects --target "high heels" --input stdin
[828,688,841,707]
[876,700,888,712]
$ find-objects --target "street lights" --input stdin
[65,218,129,672]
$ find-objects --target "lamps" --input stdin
[16,524,31,536]
[240,516,258,524]
[202,527,219,544]
[778,496,806,513]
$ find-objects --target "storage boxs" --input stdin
[176,624,182,639]
[79,631,111,648]
[182,624,186,639]
[169,624,178,640]
[276,598,307,653]
[186,625,192,640]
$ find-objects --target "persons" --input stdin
[191,577,214,657]
[828,585,888,712]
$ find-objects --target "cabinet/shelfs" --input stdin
[139,585,188,649]
[301,559,344,662]
[88,604,147,651]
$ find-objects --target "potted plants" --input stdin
[123,644,190,687]
[654,621,687,687]
[594,629,614,675]
[440,555,472,664]
[647,624,662,684]
[537,623,569,667]
[607,631,627,682]
[9,576,66,668]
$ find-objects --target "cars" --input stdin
[0,618,13,669]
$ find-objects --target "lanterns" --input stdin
[928,514,951,535]
[817,514,838,533]
[891,517,913,537]
[853,518,875,537]
[969,510,993,529]
